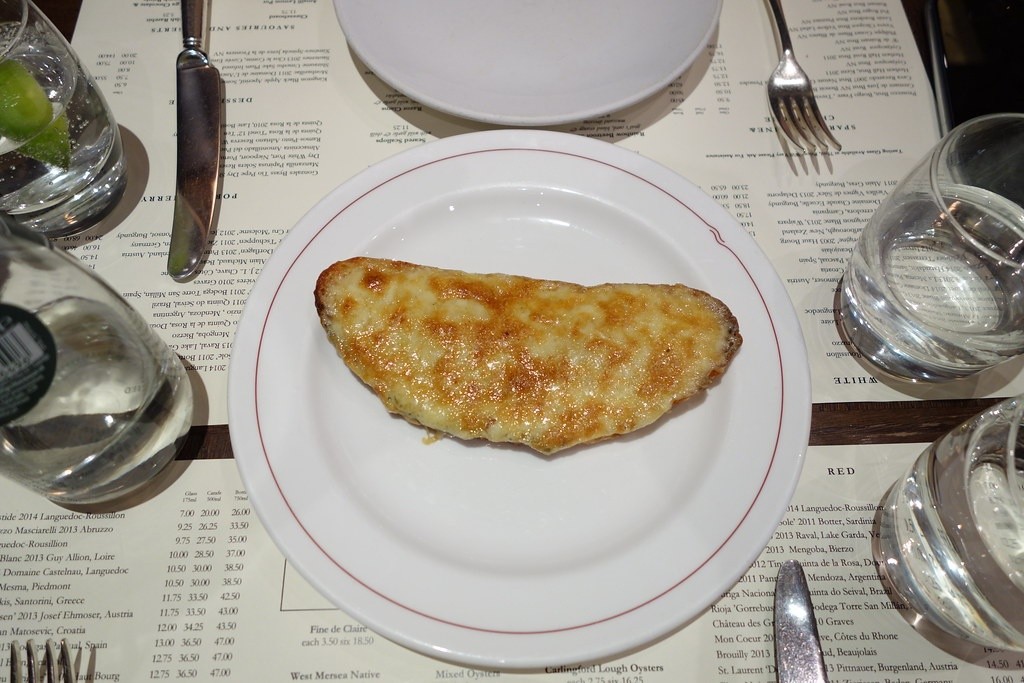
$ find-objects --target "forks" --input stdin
[9,639,73,683]
[764,0,842,155]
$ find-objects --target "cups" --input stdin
[879,391,1024,671]
[0,0,129,239]
[0,213,195,507]
[833,113,1024,386]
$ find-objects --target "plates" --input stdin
[227,126,813,672]
[332,0,721,129]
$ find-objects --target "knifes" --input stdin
[773,558,828,683]
[166,0,222,281]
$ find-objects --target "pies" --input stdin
[313,255,743,454]
